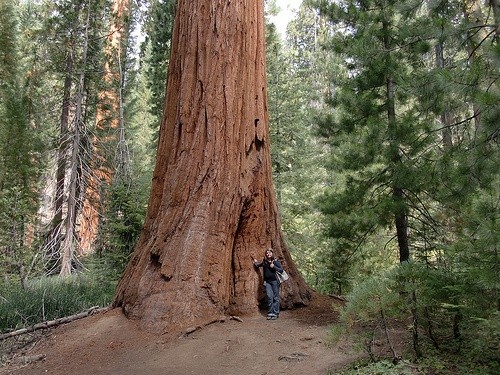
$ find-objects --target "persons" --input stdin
[252,247,284,320]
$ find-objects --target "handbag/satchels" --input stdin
[271,259,289,283]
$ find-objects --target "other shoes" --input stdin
[271,312,279,320]
[266,313,272,320]
